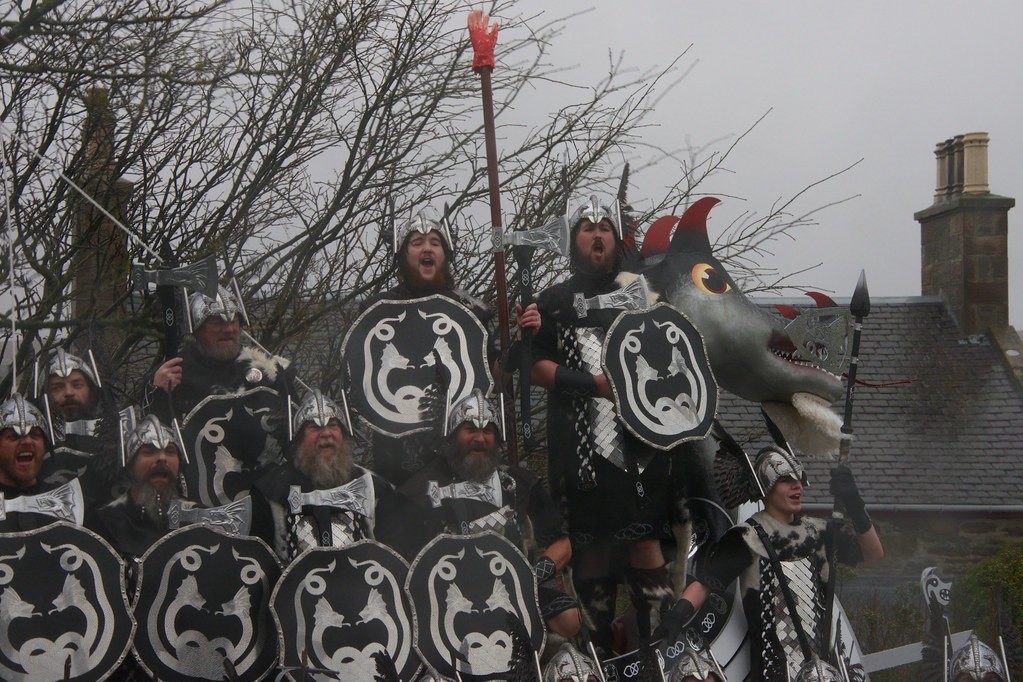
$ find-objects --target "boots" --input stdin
[576,574,618,663]
[624,564,675,655]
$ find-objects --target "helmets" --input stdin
[179,275,250,335]
[119,413,189,468]
[394,209,456,253]
[0,392,56,445]
[943,634,1012,682]
[563,193,629,243]
[287,388,354,441]
[534,641,607,682]
[439,385,507,443]
[654,648,729,682]
[745,441,812,501]
[34,347,102,398]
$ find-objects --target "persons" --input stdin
[949,633,1007,682]
[0,193,884,682]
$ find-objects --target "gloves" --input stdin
[828,466,865,514]
[649,612,686,646]
